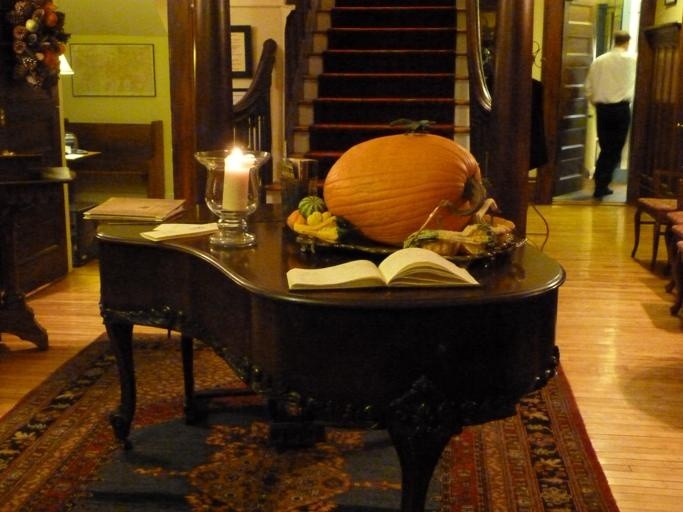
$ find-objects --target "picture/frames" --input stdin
[230,24,253,80]
[69,40,158,99]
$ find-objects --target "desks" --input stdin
[1,165,75,351]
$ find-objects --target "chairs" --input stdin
[631,166,681,315]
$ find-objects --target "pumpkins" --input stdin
[286,133,516,256]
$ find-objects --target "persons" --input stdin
[581,28,638,199]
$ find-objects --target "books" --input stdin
[285,247,481,293]
[81,195,187,223]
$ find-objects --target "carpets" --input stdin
[0,330,617,512]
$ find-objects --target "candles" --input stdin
[221,148,251,211]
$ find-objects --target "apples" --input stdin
[26,19,38,32]
